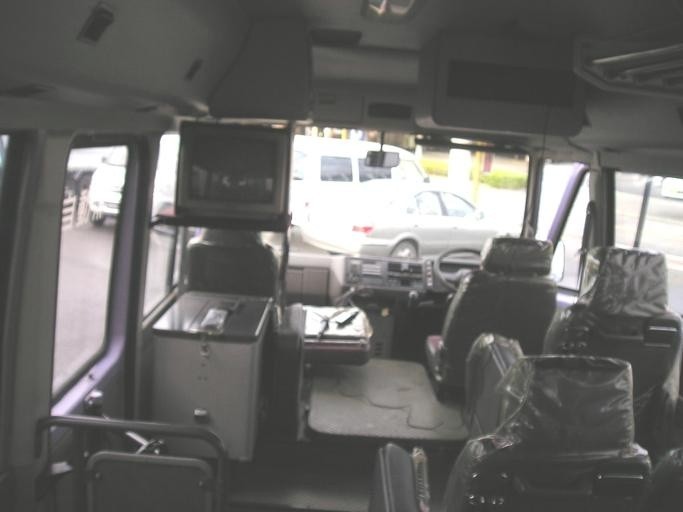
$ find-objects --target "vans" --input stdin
[293,136,428,224]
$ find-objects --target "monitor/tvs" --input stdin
[177,125,289,221]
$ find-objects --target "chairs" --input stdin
[375,355,681,509]
[463,247,681,447]
[176,224,278,297]
[424,240,557,400]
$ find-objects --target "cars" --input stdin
[302,190,498,262]
[87,136,178,223]
